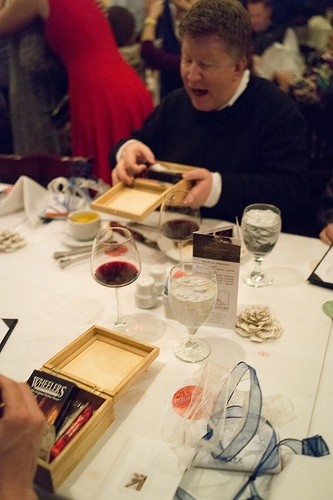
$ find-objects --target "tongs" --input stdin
[53,247,92,267]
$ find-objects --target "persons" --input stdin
[0,373,48,500]
[112,0,311,234]
[319,216,333,245]
[0,0,333,183]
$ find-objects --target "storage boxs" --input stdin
[0,325,160,494]
[91,161,196,220]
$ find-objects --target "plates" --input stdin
[56,223,97,247]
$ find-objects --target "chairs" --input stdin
[1,153,94,187]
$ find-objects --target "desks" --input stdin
[1,183,332,500]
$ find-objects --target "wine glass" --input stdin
[90,226,144,337]
[168,260,217,363]
[159,189,200,261]
[240,204,282,286]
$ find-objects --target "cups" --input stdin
[67,210,101,241]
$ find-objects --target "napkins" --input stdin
[0,175,47,225]
[46,220,114,269]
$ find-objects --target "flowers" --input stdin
[0,228,26,254]
[236,302,283,343]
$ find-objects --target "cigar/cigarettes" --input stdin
[26,368,93,459]
[141,165,182,182]
